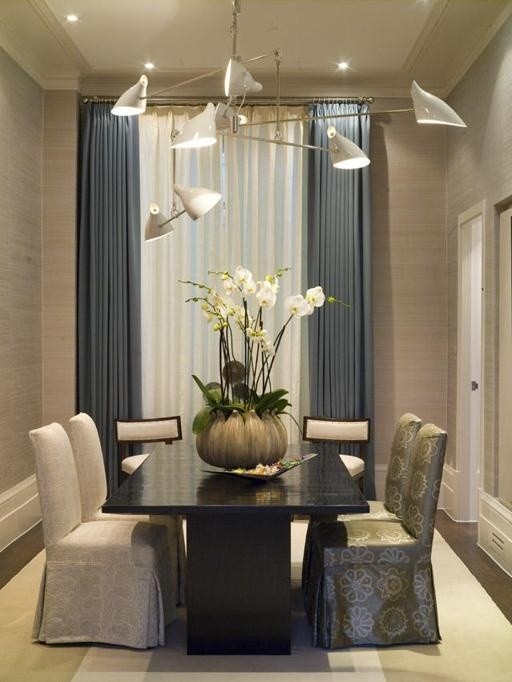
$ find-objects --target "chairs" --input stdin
[27,423,177,648]
[302,411,425,604]
[304,418,372,496]
[66,412,188,608]
[309,424,448,649]
[114,416,182,489]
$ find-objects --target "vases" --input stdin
[195,410,287,469]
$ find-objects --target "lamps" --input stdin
[110,1,467,241]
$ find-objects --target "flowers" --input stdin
[178,266,352,435]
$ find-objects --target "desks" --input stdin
[101,440,370,657]
